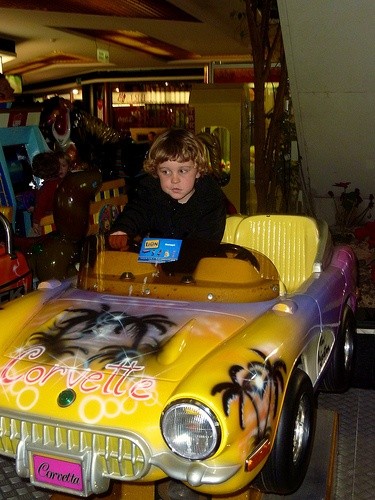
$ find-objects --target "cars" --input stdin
[0,212,357,499]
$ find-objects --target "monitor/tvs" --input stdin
[2,143,36,197]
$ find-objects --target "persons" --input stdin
[31,153,91,234]
[108,128,232,273]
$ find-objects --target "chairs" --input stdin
[220,215,334,295]
[15,168,102,289]
